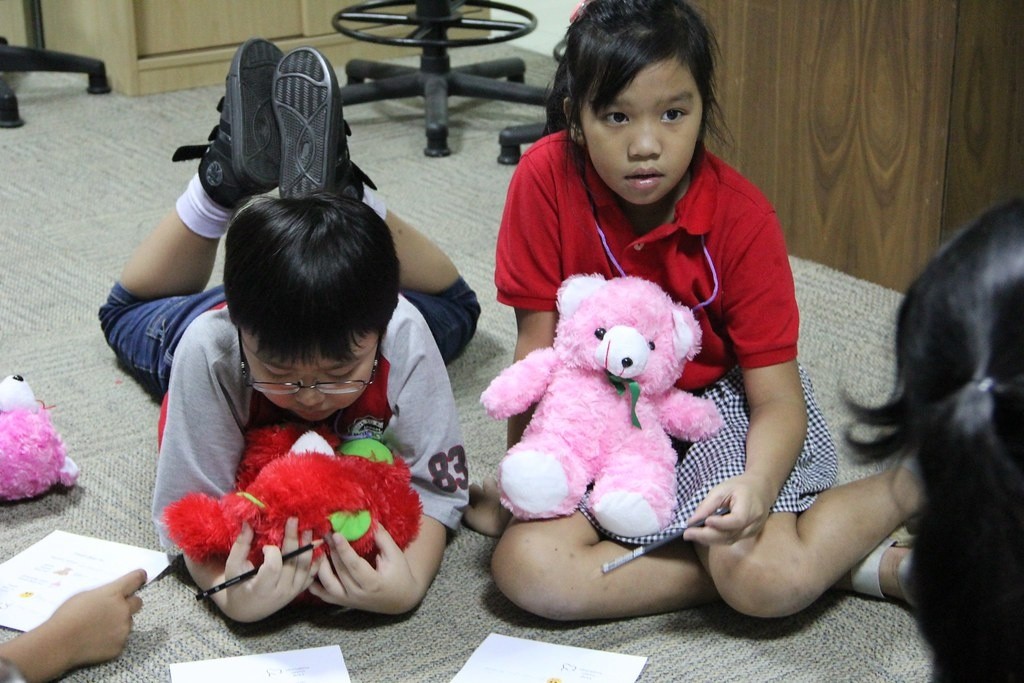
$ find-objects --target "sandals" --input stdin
[847,536,918,607]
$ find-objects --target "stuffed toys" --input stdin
[0,373,79,501]
[160,391,422,599]
[479,274,723,537]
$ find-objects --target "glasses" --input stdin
[237,329,377,394]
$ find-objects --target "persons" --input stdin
[460,0,931,619]
[845,197,1024,683]
[1,569,146,682]
[99,39,482,621]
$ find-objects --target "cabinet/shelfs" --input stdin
[0,0,491,96]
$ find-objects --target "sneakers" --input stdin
[271,45,377,202]
[171,38,282,209]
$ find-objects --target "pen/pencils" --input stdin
[600,505,731,573]
[195,537,326,603]
